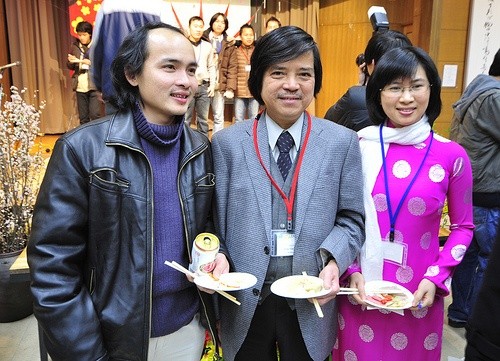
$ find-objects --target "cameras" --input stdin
[356,6,389,74]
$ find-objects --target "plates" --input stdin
[361,280,414,310]
[194,272,257,291]
[270,275,332,298]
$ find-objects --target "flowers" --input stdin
[0,72,52,254]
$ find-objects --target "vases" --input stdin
[0,234,34,324]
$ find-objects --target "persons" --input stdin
[203,13,238,135]
[88,0,174,116]
[66,21,102,125]
[235,23,260,124]
[330,44,476,361]
[26,20,237,361]
[323,31,414,133]
[184,16,216,140]
[211,25,365,361]
[228,16,282,49]
[447,48,500,361]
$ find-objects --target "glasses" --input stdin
[380,83,433,95]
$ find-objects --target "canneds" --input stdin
[192,233,220,274]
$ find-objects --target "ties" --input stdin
[277,132,295,182]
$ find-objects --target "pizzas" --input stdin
[365,292,396,307]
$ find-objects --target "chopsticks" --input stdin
[336,287,405,295]
[302,272,324,318]
[164,261,241,306]
